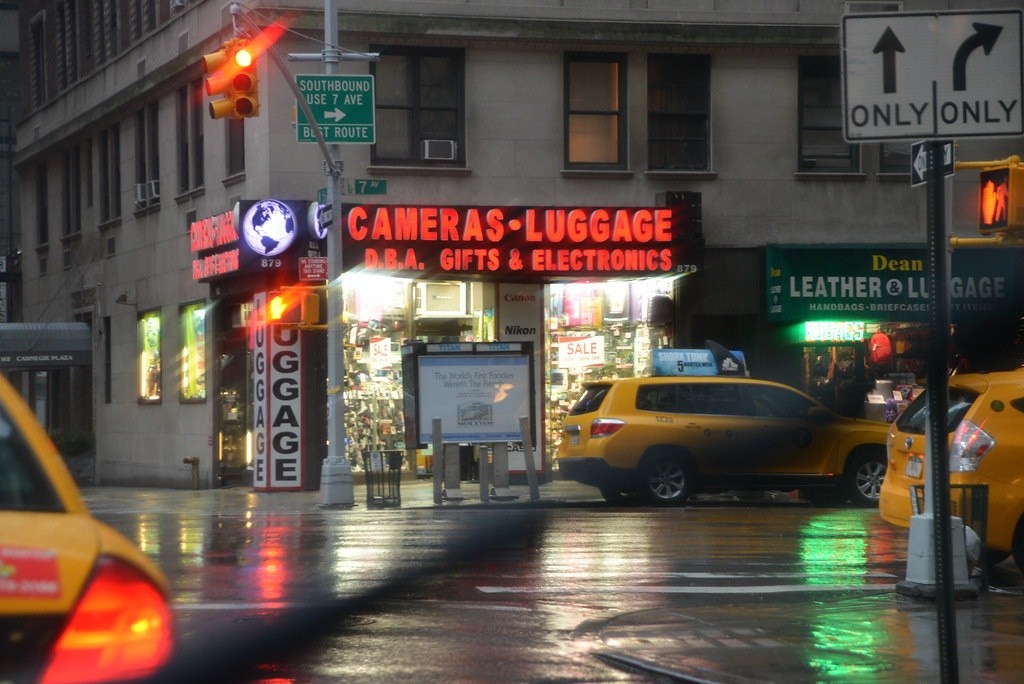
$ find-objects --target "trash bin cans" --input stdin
[359,449,404,508]
[909,481,990,587]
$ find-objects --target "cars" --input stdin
[556,375,898,508]
[877,364,1023,574]
[0,372,180,683]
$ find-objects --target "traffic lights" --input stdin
[973,161,1016,236]
[200,35,263,123]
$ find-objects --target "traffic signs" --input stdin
[294,72,378,146]
[840,7,1024,144]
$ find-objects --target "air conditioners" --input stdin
[147,180,159,202]
[134,183,146,205]
[420,139,458,163]
